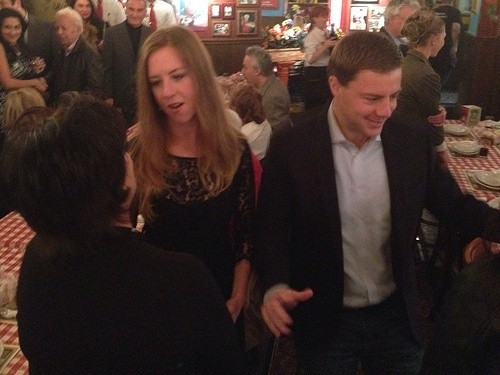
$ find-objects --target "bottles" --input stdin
[329,24,337,54]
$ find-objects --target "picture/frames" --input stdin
[349,6,368,31]
[221,2,235,20]
[210,3,221,18]
[351,0,379,4]
[235,0,261,8]
[212,21,232,37]
[237,10,258,38]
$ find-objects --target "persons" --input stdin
[0,0,499,375]
[128,26,255,355]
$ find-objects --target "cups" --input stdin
[234,73,245,83]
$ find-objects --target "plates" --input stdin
[443,124,470,136]
[447,141,482,157]
[473,170,500,191]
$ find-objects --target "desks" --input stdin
[416,118,500,268]
[0,210,143,375]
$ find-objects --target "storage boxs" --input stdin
[458,103,482,126]
